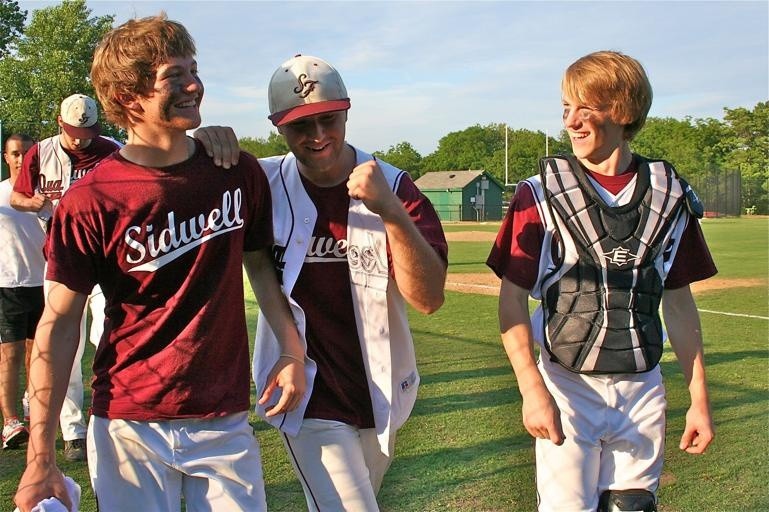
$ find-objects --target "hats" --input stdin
[60,94,101,139]
[268,52,350,125]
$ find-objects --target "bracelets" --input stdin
[278,352,307,366]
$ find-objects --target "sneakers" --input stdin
[1,420,29,451]
[65,438,87,461]
[22,397,30,421]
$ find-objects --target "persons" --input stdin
[15,10,309,512]
[9,95,123,464]
[484,48,722,510]
[2,135,48,451]
[195,54,451,512]
